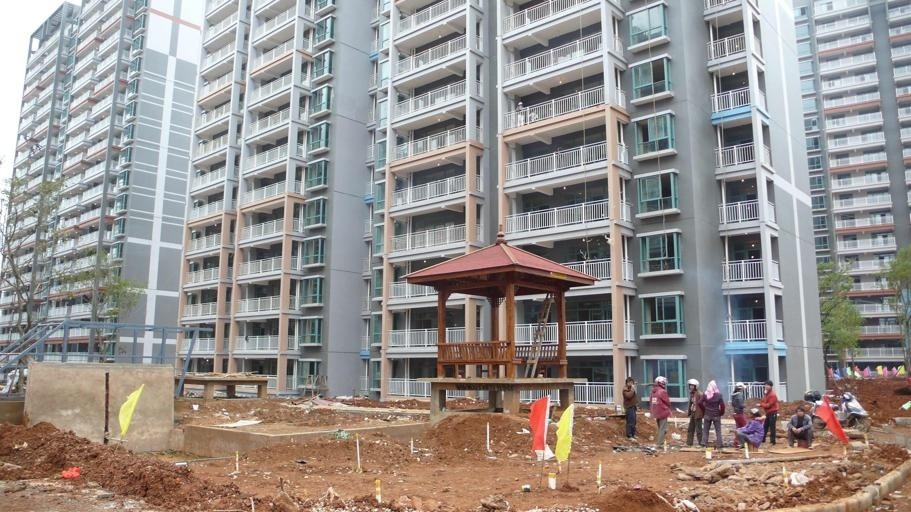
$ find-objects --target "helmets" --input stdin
[655,376,667,385]
[688,379,699,390]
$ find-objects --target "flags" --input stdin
[118,385,142,439]
[528,394,551,457]
[815,400,850,448]
[827,364,905,380]
[552,403,575,462]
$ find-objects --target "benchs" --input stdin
[438,342,567,377]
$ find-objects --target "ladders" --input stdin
[523,293,553,378]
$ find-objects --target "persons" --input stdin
[756,379,780,446]
[622,376,641,442]
[786,407,813,449]
[729,382,748,448]
[700,379,726,447]
[647,376,672,445]
[680,377,705,447]
[730,407,766,449]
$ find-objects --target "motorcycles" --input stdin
[803,388,873,431]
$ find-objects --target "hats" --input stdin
[736,383,747,389]
